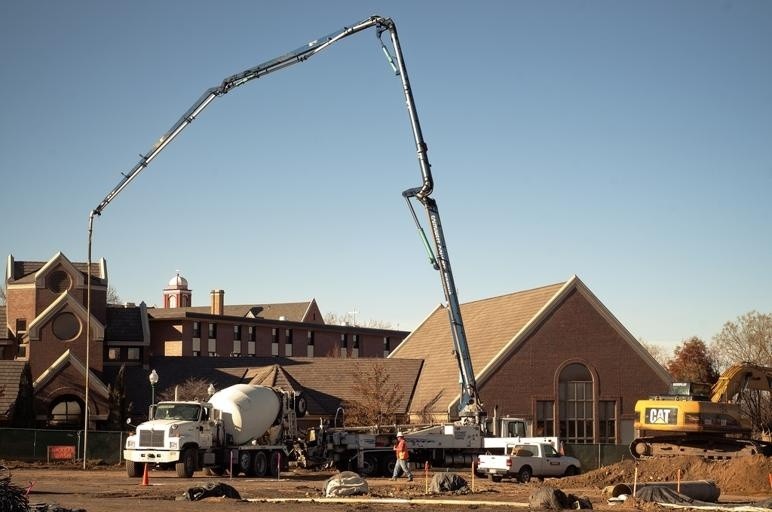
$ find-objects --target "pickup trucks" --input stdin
[476,442,581,482]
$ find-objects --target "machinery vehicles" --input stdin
[628,364,772,459]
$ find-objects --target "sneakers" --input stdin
[406,476,413,482]
[389,476,397,481]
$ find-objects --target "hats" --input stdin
[396,432,404,437]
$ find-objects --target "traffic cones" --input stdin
[140,462,150,486]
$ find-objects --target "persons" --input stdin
[389,432,413,482]
